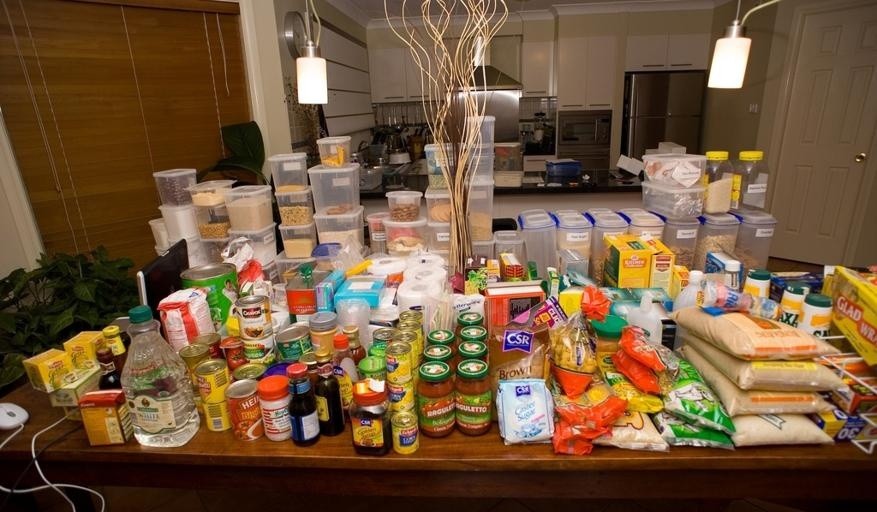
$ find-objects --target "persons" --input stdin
[221,278,239,305]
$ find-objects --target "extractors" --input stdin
[440,35,522,93]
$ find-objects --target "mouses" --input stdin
[0,403,29,430]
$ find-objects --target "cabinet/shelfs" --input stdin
[366,42,444,102]
[624,33,708,72]
[522,40,554,97]
[555,37,614,112]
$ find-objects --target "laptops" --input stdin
[109,238,189,330]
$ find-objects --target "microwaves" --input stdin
[558,110,610,145]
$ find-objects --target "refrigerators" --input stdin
[624,71,706,159]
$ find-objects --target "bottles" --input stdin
[700,150,734,215]
[95,325,125,390]
[672,269,702,350]
[117,305,201,449]
[282,324,365,447]
[730,151,770,215]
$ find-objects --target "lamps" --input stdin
[706,2,780,91]
[297,0,328,105]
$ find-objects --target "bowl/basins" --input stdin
[388,153,411,165]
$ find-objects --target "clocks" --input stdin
[284,11,306,58]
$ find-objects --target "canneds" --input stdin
[178,294,492,456]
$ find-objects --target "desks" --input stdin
[1,306,876,511]
